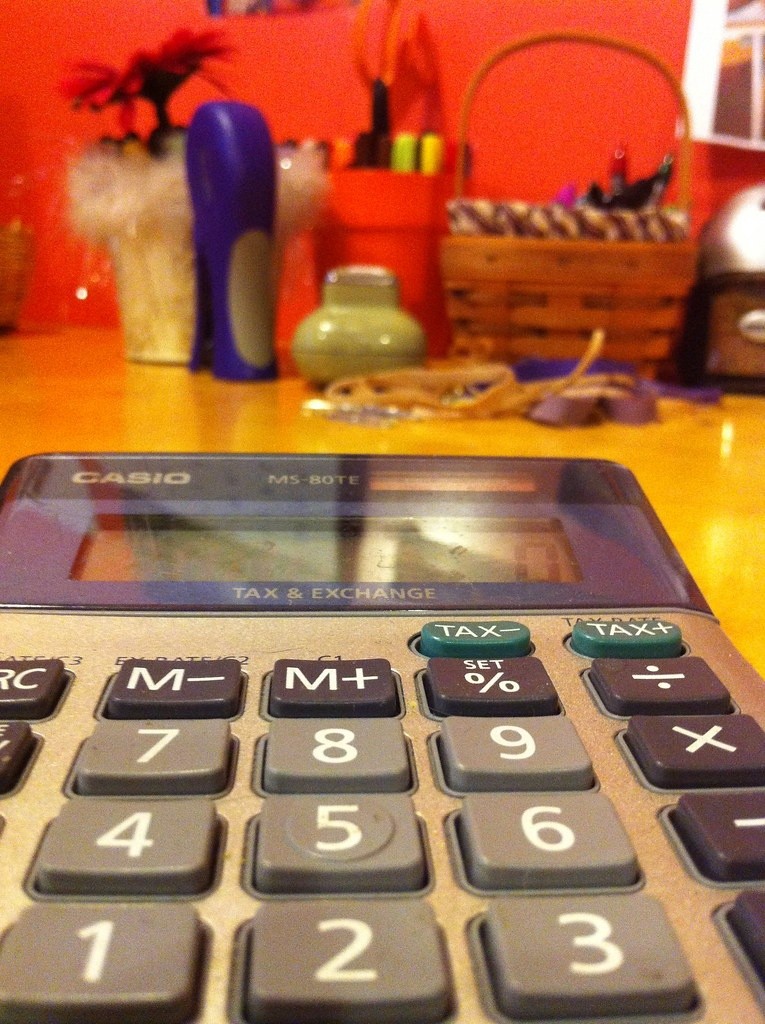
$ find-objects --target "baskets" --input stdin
[437,27,699,381]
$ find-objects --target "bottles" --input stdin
[291,265,425,385]
[184,99,285,378]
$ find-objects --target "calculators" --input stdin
[0,443,765,1024]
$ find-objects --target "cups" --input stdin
[321,169,435,313]
